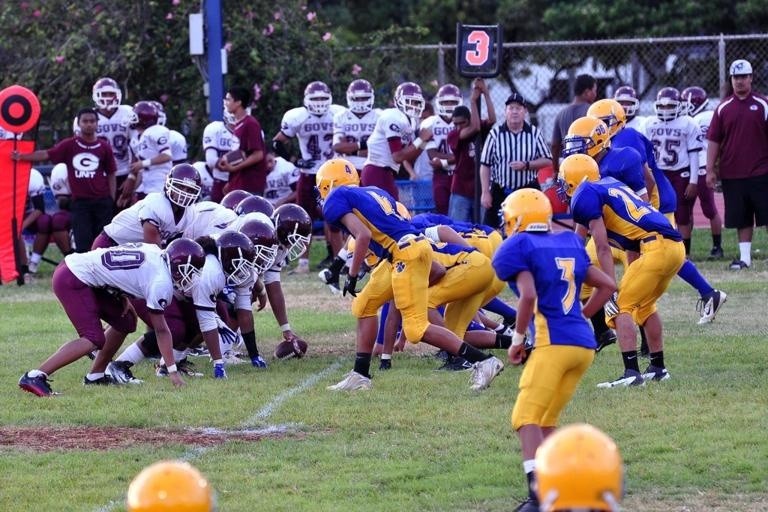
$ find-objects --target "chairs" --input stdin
[22,189,71,272]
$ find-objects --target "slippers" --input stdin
[729,259,751,270]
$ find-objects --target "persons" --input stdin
[492,187,616,508]
[10,58,768,398]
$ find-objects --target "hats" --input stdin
[729,59,753,76]
[505,93,528,108]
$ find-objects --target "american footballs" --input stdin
[272,341,307,361]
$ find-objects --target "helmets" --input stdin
[223,98,254,127]
[346,236,373,261]
[392,81,426,119]
[561,115,612,159]
[654,86,682,123]
[313,157,360,210]
[434,83,463,120]
[120,100,158,130]
[270,202,314,262]
[234,195,276,220]
[163,162,202,208]
[302,80,333,116]
[586,97,628,139]
[555,152,601,206]
[216,229,258,291]
[150,100,167,125]
[614,84,640,118]
[164,237,206,294]
[497,187,555,241]
[91,77,122,112]
[237,219,279,276]
[345,78,375,114]
[681,85,710,118]
[219,189,254,210]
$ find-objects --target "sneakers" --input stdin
[17,371,65,399]
[595,328,620,352]
[467,353,505,392]
[637,348,651,360]
[27,259,39,279]
[512,497,540,512]
[638,364,671,383]
[85,350,100,361]
[285,265,310,277]
[520,337,534,365]
[325,368,375,394]
[153,356,206,378]
[104,359,147,386]
[184,343,211,358]
[695,288,728,326]
[82,373,127,389]
[596,368,646,390]
[316,266,342,296]
[433,355,474,374]
[501,316,519,329]
[706,245,725,261]
[494,324,514,338]
[208,353,249,366]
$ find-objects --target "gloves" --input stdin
[324,258,344,285]
[251,355,268,370]
[342,272,358,298]
[602,291,620,318]
[211,358,228,380]
[377,353,391,372]
[219,286,237,306]
